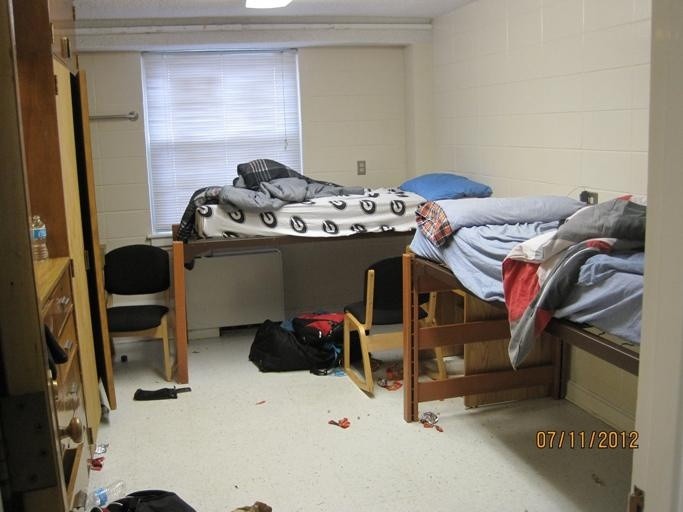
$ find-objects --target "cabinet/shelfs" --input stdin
[0,1,100,512]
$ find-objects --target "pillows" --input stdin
[398,173,492,200]
[413,195,586,248]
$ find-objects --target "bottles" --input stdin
[86,481,127,506]
[29,214,48,263]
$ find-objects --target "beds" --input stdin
[398,195,645,423]
[171,184,424,389]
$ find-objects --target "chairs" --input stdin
[103,243,173,385]
[343,256,448,394]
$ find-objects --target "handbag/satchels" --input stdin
[247,309,361,373]
